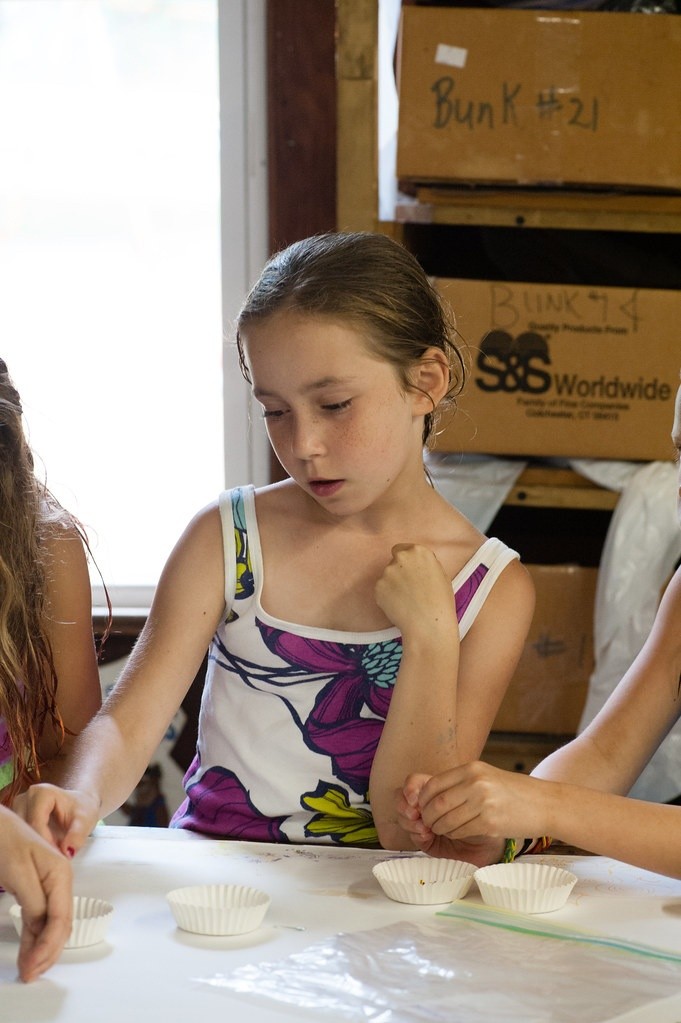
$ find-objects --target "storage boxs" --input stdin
[465,563,597,773]
[396,1,680,462]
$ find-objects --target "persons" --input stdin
[0,355,102,983]
[393,383,681,883]
[9,233,535,869]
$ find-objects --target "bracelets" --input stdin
[498,834,553,866]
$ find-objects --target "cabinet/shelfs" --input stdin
[393,204,681,509]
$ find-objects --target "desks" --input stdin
[0,828,681,1023]
